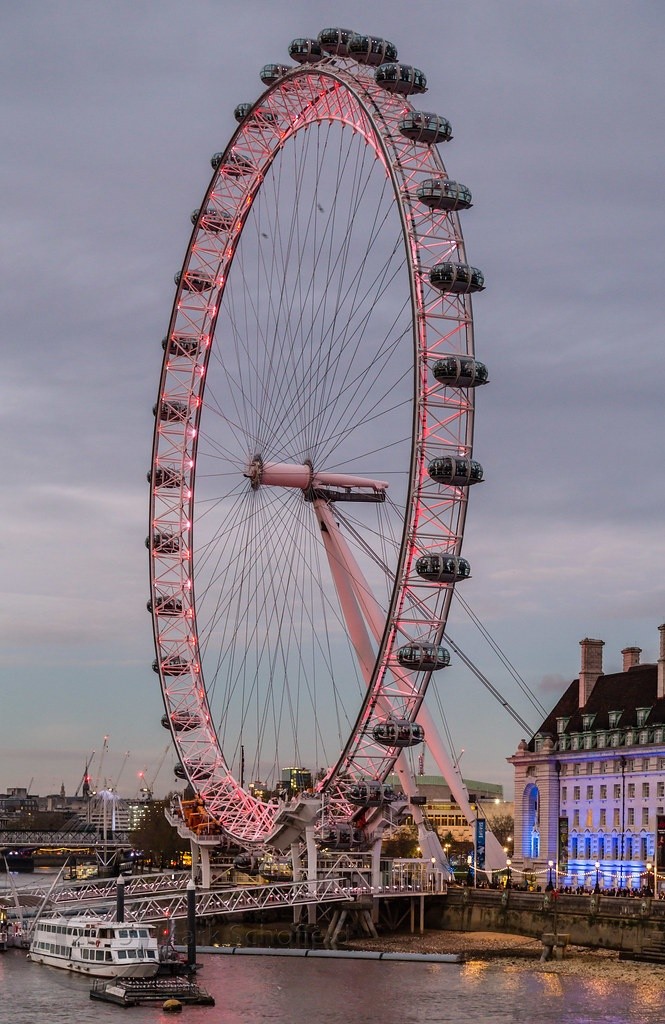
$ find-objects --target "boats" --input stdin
[26,909,161,978]
[0,855,33,950]
[155,912,203,977]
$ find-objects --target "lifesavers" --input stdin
[95,940,101,947]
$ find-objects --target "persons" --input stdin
[560,883,654,898]
[444,877,475,888]
[476,877,541,892]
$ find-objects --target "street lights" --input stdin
[646,861,652,896]
[594,859,600,894]
[547,858,554,891]
[443,843,451,859]
[506,858,512,889]
